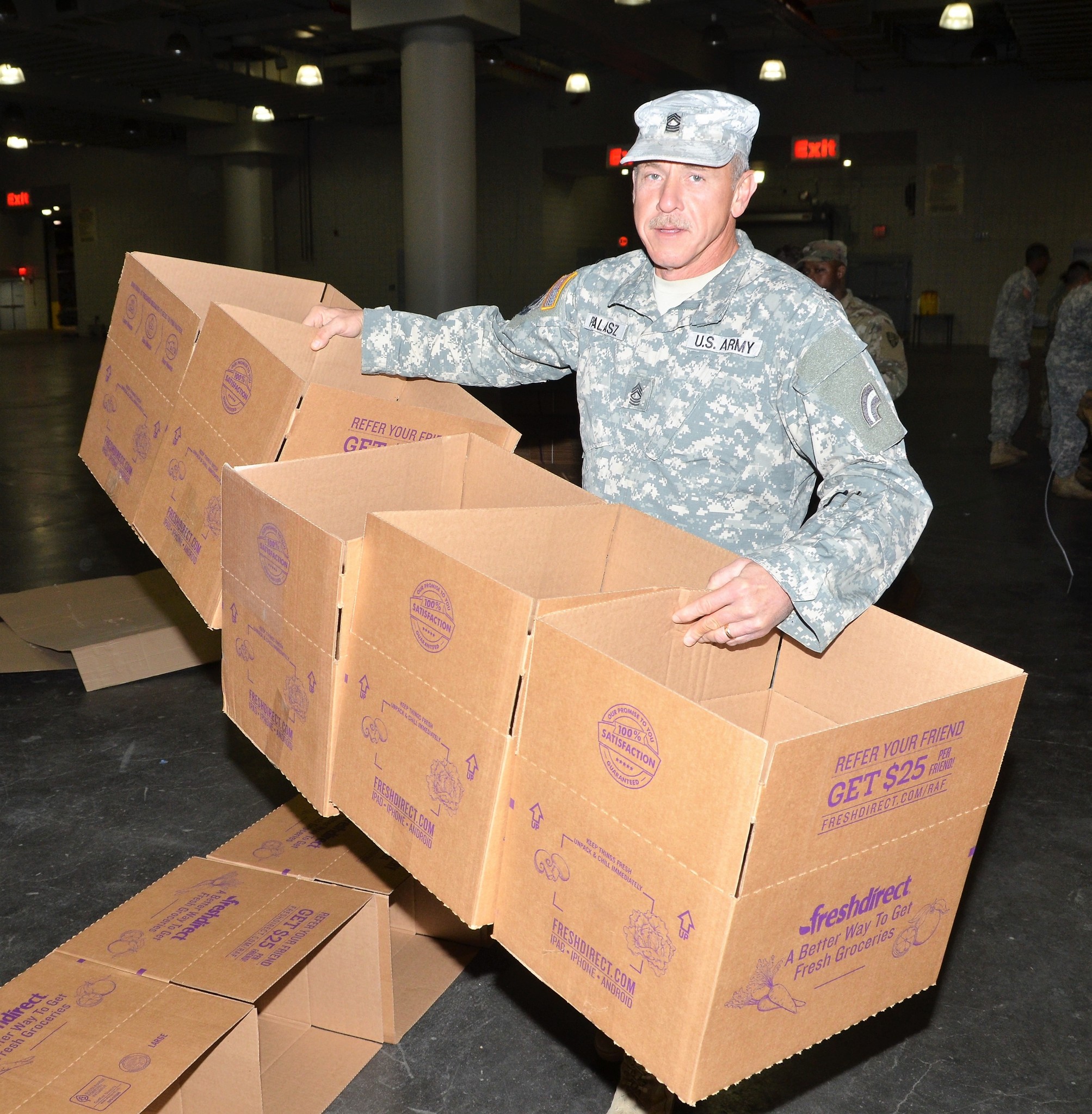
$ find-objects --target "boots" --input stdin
[989,435,1018,467]
[1075,461,1092,482]
[1051,472,1092,501]
[1006,437,1031,460]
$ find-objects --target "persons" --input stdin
[1044,251,1092,496]
[798,240,909,397]
[302,88,933,658]
[985,240,1046,474]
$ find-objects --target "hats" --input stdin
[620,90,759,167]
[795,240,847,268]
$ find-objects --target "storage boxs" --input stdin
[0,249,1026,1114]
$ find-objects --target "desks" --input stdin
[912,313,953,347]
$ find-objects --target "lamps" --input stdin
[252,103,274,121]
[0,64,25,85]
[565,71,591,94]
[759,58,786,81]
[939,0,974,30]
[296,58,322,87]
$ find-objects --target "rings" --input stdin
[724,623,735,640]
